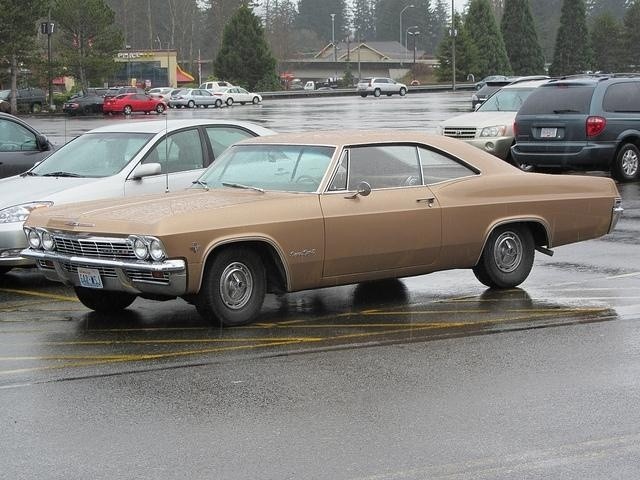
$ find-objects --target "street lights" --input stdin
[41,21,57,115]
[125,43,133,83]
[408,31,420,63]
[330,13,337,62]
[399,5,414,66]
[405,25,418,59]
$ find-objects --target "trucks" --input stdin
[304,80,338,89]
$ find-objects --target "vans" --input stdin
[282,79,302,89]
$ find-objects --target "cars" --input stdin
[0,119,348,276]
[18,128,626,325]
[474,75,507,89]
[0,81,262,116]
[0,108,63,179]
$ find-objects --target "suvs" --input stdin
[471,75,551,110]
[511,73,640,183]
[436,76,562,173]
[356,76,408,96]
